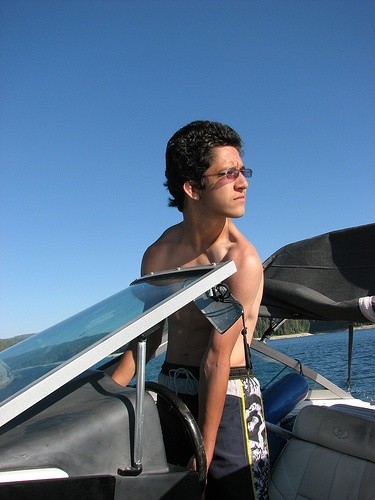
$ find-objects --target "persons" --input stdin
[111,119,271,500]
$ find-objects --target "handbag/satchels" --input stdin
[262,371,308,424]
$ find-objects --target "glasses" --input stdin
[191,167,253,181]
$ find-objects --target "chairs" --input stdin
[268,404,375,500]
[262,372,309,424]
